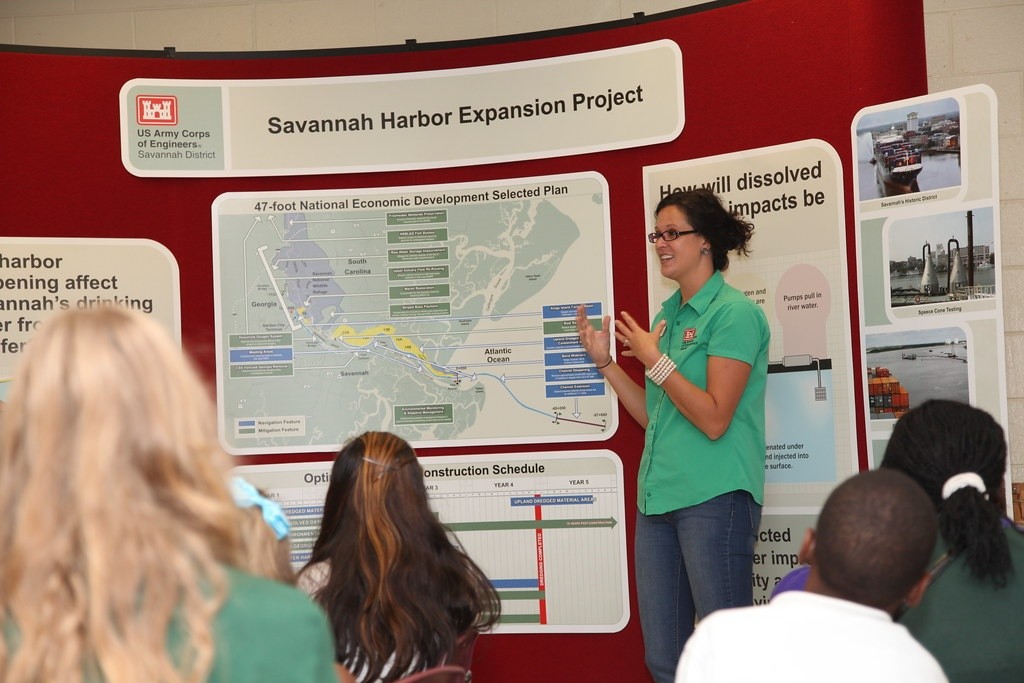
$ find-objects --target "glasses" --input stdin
[648,229,698,243]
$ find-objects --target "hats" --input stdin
[230,477,292,541]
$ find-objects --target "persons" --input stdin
[675,470,948,683]
[0,306,337,683]
[292,433,501,683]
[576,189,771,683]
[878,399,1023,683]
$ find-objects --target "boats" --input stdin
[977,262,995,271]
[872,133,923,190]
[902,353,917,360]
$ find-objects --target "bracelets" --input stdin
[623,339,629,346]
[645,353,677,386]
[596,356,612,369]
